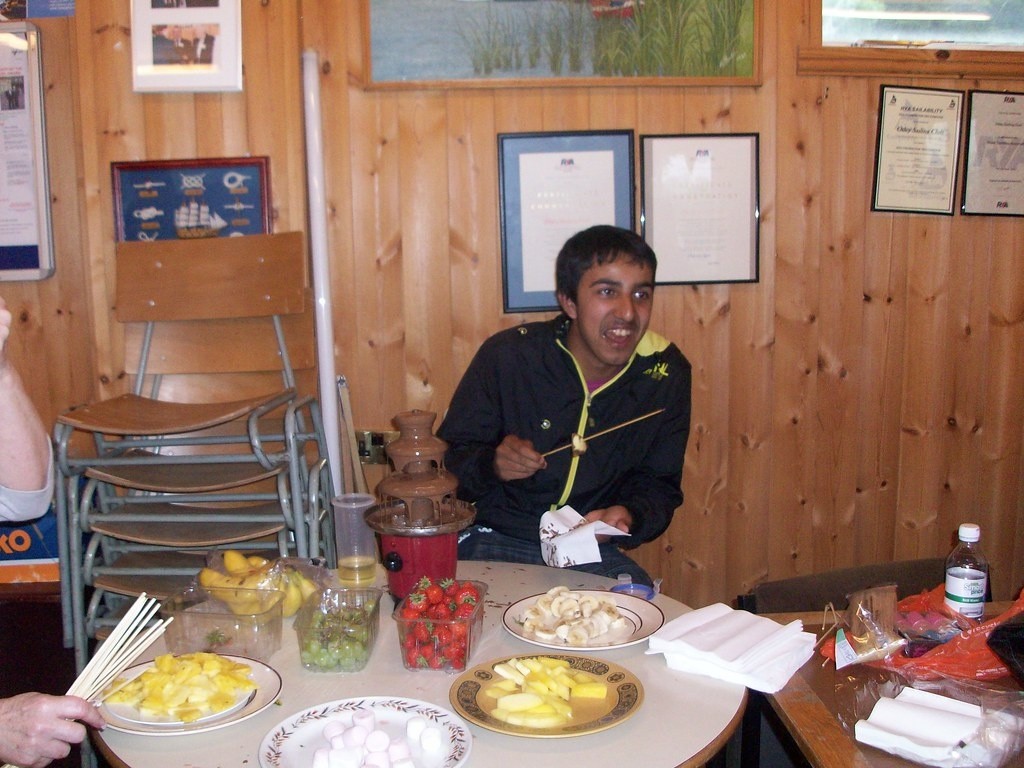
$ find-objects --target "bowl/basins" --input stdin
[154,586,287,664]
[292,585,384,674]
[391,577,491,672]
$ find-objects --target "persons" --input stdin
[433,225,691,590]
[0,296,109,768]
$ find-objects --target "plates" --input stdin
[500,588,667,652]
[93,653,283,737]
[258,695,474,767]
[106,666,254,728]
[448,651,647,739]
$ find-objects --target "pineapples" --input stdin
[104,651,257,723]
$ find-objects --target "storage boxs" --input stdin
[292,587,385,674]
[391,578,489,675]
[156,585,286,663]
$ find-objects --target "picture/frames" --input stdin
[960,87,1024,218]
[638,131,764,285]
[130,0,245,94]
[359,0,764,92]
[109,156,274,243]
[869,84,965,218]
[496,127,636,315]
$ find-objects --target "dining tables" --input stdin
[755,598,1024,768]
[83,559,750,768]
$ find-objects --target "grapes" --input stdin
[299,598,380,672]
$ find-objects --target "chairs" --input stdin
[736,558,994,768]
[51,232,337,768]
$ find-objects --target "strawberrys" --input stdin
[397,575,482,669]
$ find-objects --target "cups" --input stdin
[330,493,378,587]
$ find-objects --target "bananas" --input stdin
[523,585,627,643]
[200,550,320,621]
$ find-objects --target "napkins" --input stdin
[853,686,1024,768]
[644,600,818,693]
[539,505,632,568]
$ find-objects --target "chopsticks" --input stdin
[2,591,173,768]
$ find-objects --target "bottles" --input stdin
[943,523,989,625]
